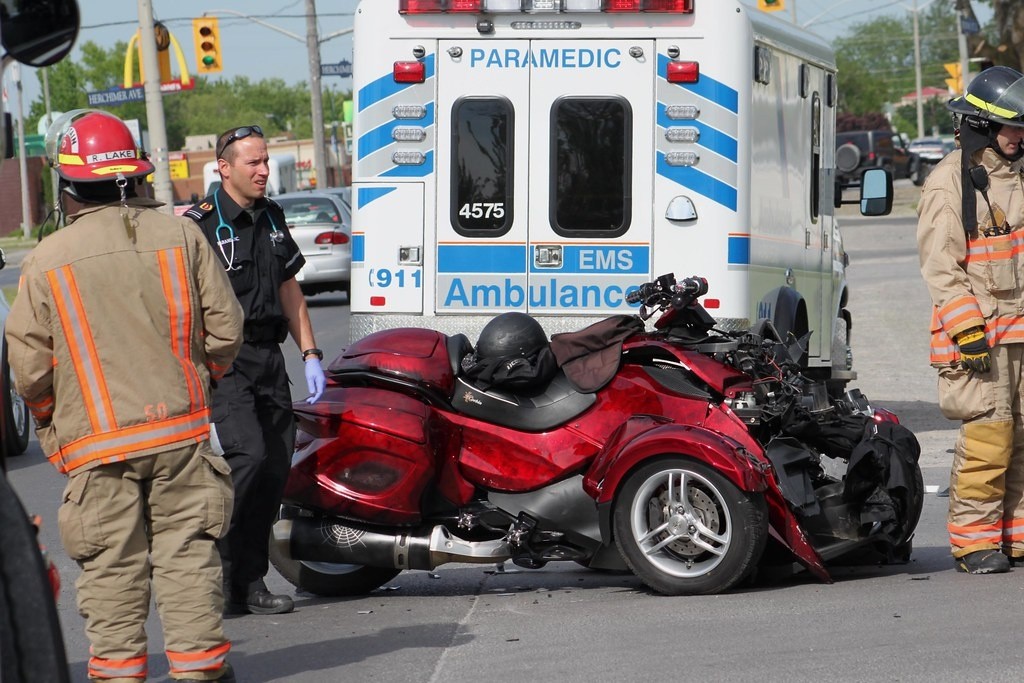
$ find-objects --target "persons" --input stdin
[916,66,1024,576]
[6,109,244,683]
[183,126,326,614]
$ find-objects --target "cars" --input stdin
[907,136,957,160]
[268,184,351,303]
[0,247,33,456]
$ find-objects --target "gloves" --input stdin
[956,326,992,373]
[304,358,326,405]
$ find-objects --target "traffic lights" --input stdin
[758,0,785,11]
[944,60,964,97]
[192,18,222,73]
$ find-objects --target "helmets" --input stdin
[55,112,155,183]
[947,65,1024,128]
[474,313,549,361]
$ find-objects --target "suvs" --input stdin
[835,129,924,190]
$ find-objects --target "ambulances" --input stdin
[346,0,895,405]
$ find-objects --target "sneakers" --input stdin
[955,548,1010,574]
[239,588,293,613]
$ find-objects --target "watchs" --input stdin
[301,348,323,361]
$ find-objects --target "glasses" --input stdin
[218,125,263,159]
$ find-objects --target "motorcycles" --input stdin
[261,272,926,599]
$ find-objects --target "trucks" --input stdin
[201,153,301,200]
